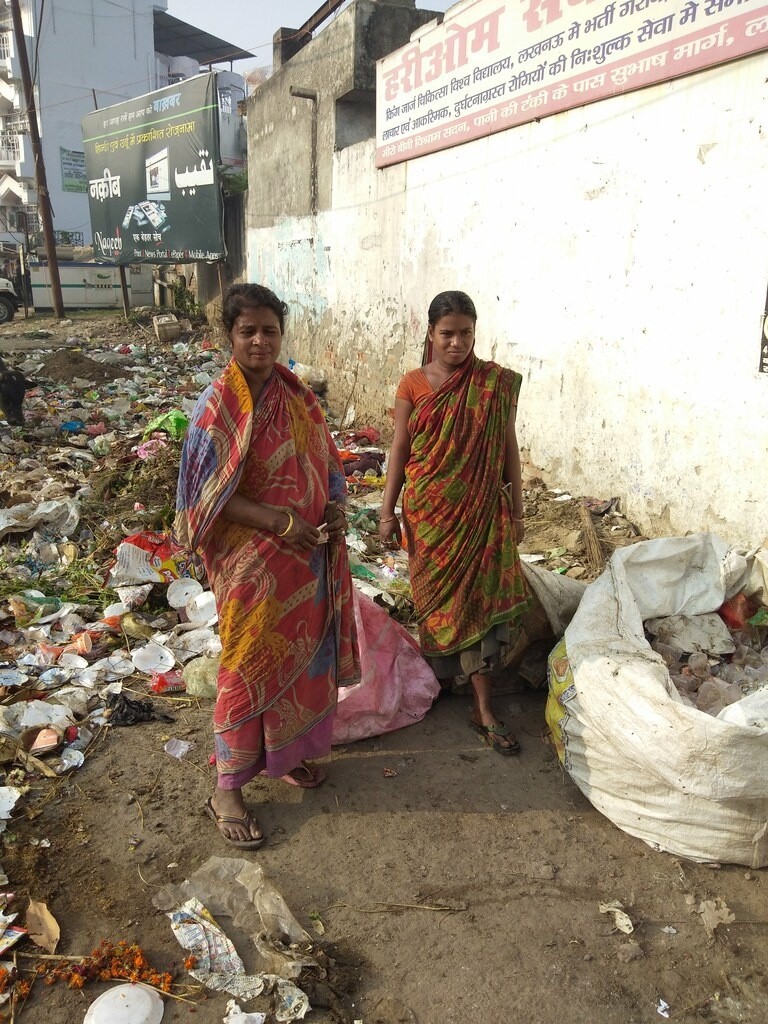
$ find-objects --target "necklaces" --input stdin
[437,358,457,371]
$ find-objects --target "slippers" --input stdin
[471,716,520,755]
[204,795,266,850]
[258,760,326,788]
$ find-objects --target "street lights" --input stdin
[15,211,30,255]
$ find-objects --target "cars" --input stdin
[0,277,19,325]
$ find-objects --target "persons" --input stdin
[378,291,533,755]
[170,282,363,850]
[0,258,31,312]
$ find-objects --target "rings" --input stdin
[380,540,394,544]
[339,527,346,537]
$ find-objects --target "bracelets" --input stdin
[275,511,294,538]
[379,513,396,523]
[512,512,526,522]
